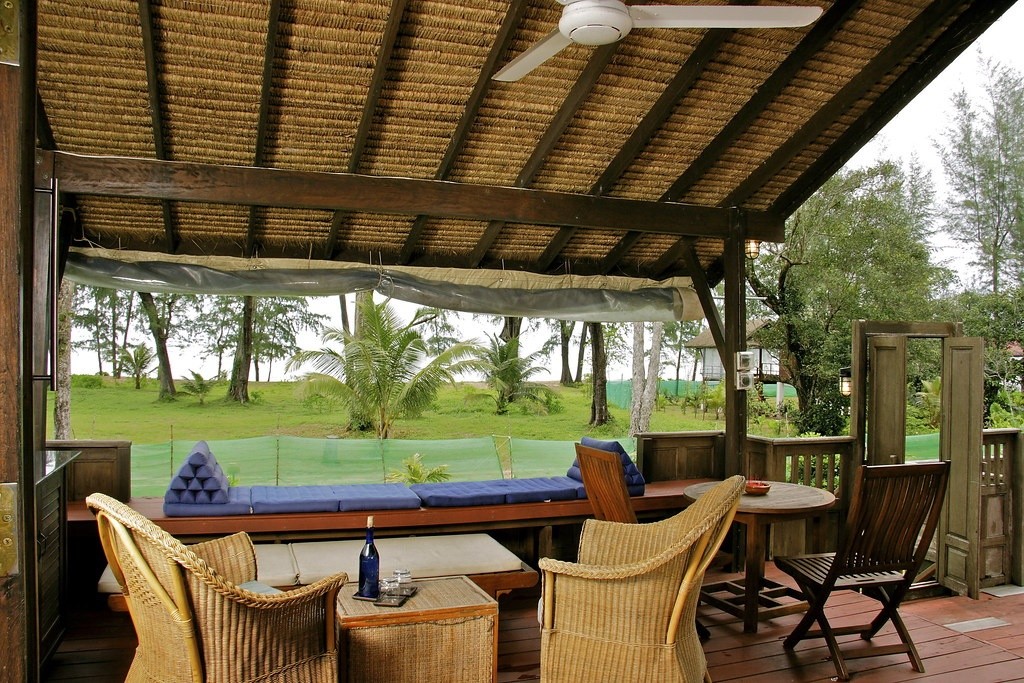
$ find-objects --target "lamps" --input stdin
[839,367,851,397]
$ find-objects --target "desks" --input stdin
[333,574,499,683]
[683,481,835,635]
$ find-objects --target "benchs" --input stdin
[97,531,538,600]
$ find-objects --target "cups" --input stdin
[379,577,400,603]
[392,568,412,594]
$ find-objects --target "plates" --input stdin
[386,586,417,596]
[351,591,385,601]
[373,596,406,607]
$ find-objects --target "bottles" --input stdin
[358,515,380,598]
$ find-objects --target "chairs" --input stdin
[773,460,952,681]
[85,491,350,683]
[538,475,746,683]
[576,442,714,640]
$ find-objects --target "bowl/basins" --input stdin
[745,482,771,495]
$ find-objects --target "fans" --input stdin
[492,0,823,83]
